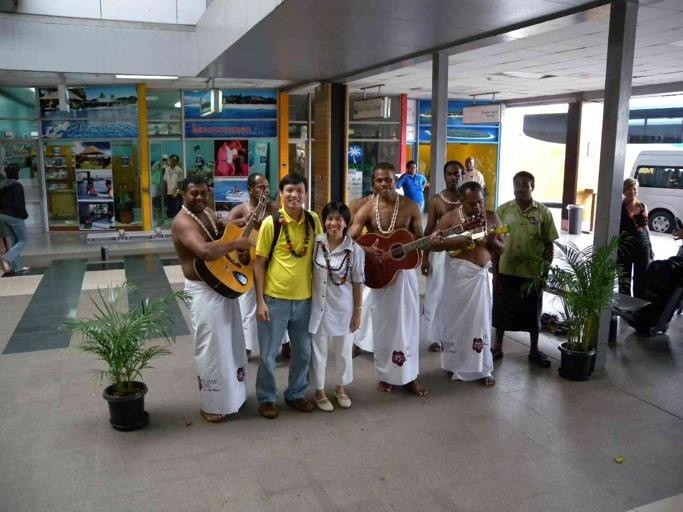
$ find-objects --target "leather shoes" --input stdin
[22,266,29,271]
[312,392,334,411]
[1,258,9,272]
[258,402,277,418]
[334,389,351,409]
[285,396,314,412]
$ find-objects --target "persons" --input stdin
[162,155,185,222]
[216,140,247,175]
[346,157,559,397]
[0,172,12,253]
[95,178,113,198]
[88,185,98,195]
[171,172,365,423]
[614,176,655,297]
[186,156,214,187]
[0,162,32,272]
[23,146,38,178]
[672,227,683,241]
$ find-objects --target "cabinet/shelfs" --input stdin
[43,145,76,219]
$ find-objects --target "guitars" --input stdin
[194,189,269,298]
[444,225,511,257]
[356,213,486,288]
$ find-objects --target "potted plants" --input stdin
[60,278,193,433]
[518,231,632,381]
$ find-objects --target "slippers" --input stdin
[409,380,427,396]
[484,376,496,387]
[379,382,391,392]
[429,343,440,351]
[199,410,228,422]
[529,349,546,359]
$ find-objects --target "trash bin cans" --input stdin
[568,206,583,234]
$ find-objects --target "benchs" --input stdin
[543,270,652,342]
[86,227,174,262]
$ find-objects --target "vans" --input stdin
[626,149,682,234]
